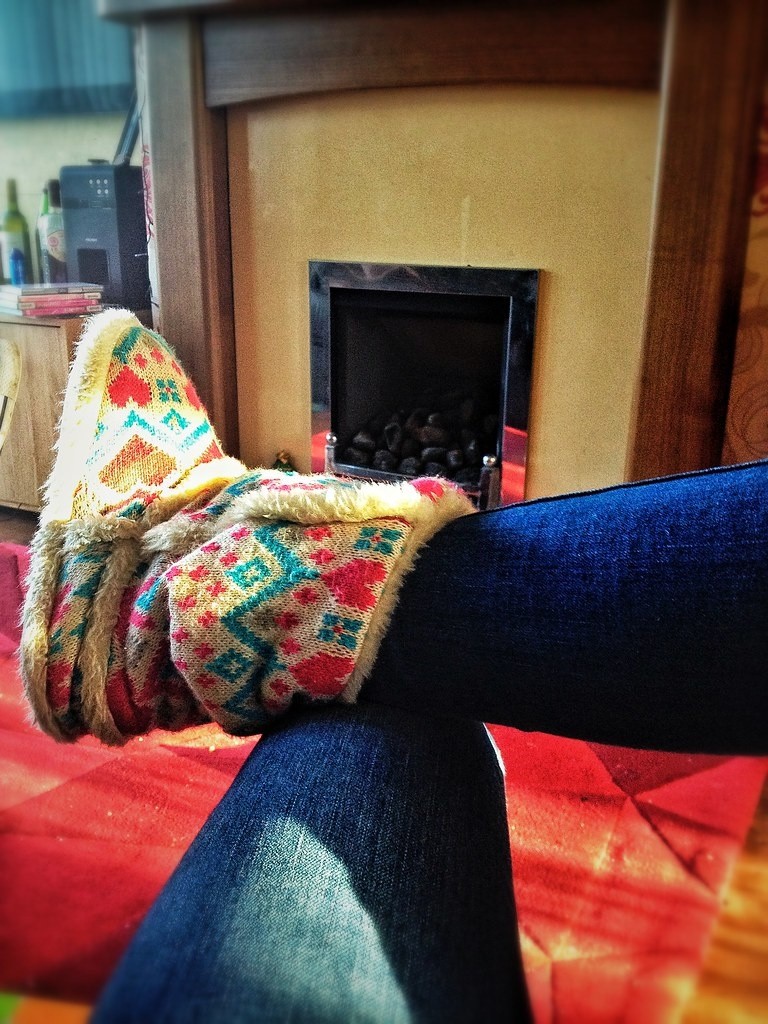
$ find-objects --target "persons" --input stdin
[18,307,768,1024]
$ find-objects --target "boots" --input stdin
[20,305,470,743]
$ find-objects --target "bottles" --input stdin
[1,178,32,285]
[10,247,26,286]
[42,179,67,283]
[35,186,49,284]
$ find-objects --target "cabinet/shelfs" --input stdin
[0,308,153,516]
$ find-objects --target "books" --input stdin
[0,282,104,317]
